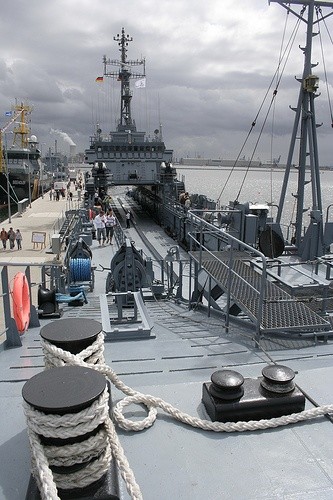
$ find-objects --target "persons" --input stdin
[125,209,131,229]
[14,229,23,250]
[105,210,116,245]
[94,210,108,247]
[165,185,209,218]
[49,176,82,201]
[90,174,112,238]
[7,227,16,251]
[0,228,7,249]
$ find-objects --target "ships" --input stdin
[0,0,333,500]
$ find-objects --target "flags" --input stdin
[96,77,103,83]
[135,78,145,88]
[5,111,12,117]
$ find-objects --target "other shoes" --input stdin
[98,239,113,247]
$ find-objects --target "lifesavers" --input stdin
[11,274,34,331]
